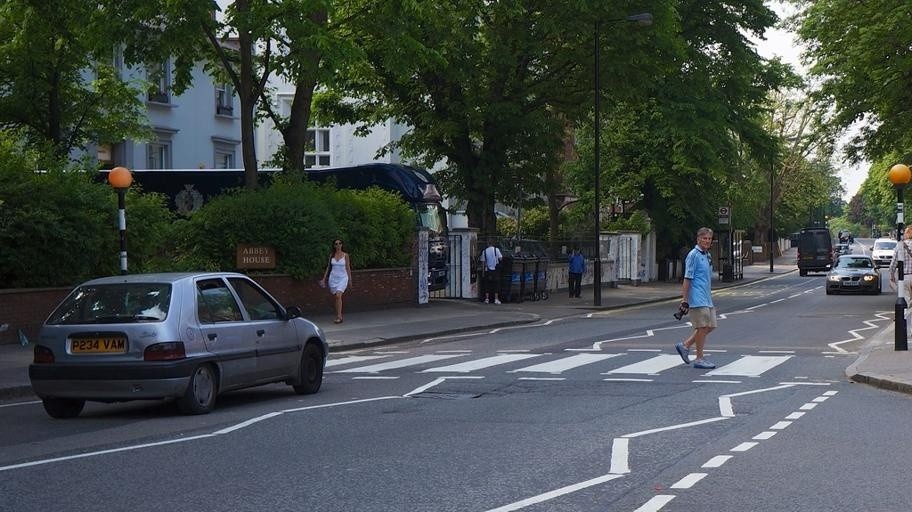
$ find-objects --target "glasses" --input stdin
[335,242,341,245]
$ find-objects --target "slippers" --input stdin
[334,317,343,323]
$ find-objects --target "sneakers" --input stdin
[694,360,715,369]
[494,299,501,304]
[675,342,690,364]
[483,299,489,304]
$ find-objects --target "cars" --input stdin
[836,229,854,257]
[27,270,334,421]
[823,255,884,296]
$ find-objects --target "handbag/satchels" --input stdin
[487,263,502,282]
[327,255,334,281]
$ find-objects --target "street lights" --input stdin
[590,12,655,308]
[105,164,136,275]
[886,162,911,352]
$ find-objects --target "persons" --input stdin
[887,223,912,345]
[479,238,504,305]
[673,225,718,371]
[317,237,355,325]
[566,245,586,298]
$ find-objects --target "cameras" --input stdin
[673,302,689,320]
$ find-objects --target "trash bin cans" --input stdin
[498,251,549,303]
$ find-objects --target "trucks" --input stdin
[25,153,452,291]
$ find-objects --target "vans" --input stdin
[796,226,838,278]
[869,237,897,268]
